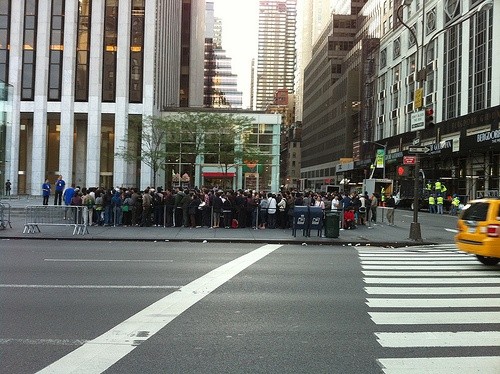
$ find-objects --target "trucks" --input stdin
[321,185,341,194]
[362,178,393,206]
[394,176,447,212]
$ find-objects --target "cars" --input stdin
[454,198,500,265]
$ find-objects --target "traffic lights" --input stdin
[424,107,435,131]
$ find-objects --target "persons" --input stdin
[61,185,397,230]
[53,175,65,205]
[5,180,11,196]
[426,179,464,216]
[41,178,51,205]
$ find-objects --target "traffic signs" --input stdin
[408,145,432,155]
[412,136,422,146]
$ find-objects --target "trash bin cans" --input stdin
[292,206,309,238]
[308,206,323,238]
[325,213,340,239]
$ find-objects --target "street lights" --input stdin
[397,0,494,241]
[362,139,388,179]
